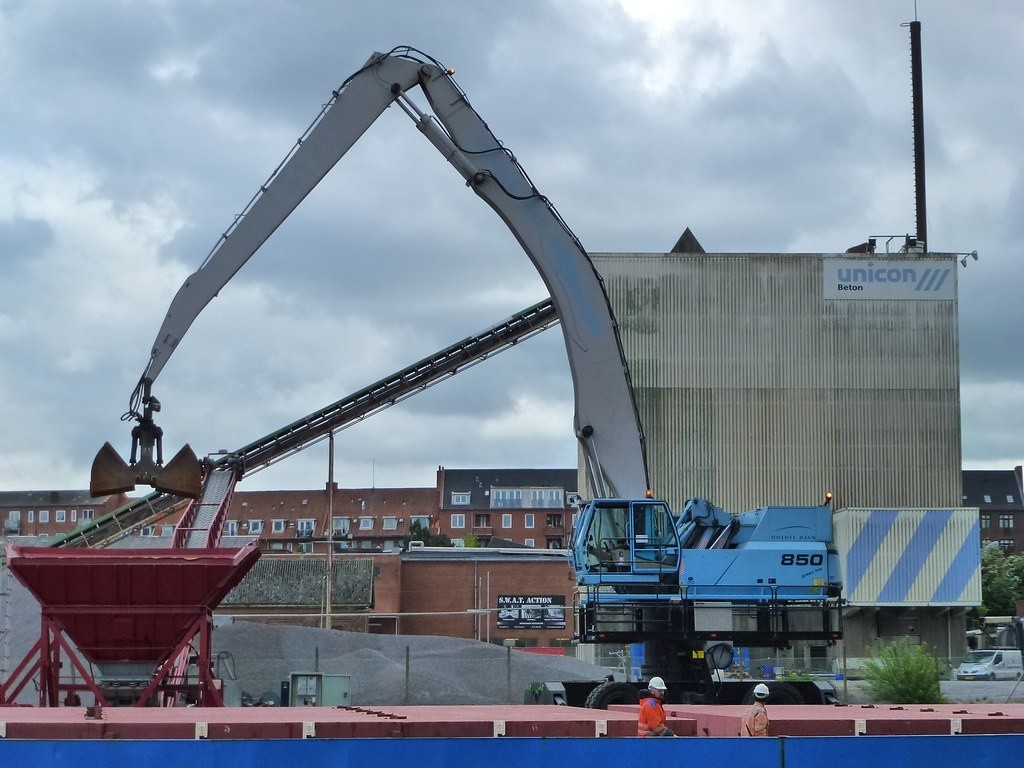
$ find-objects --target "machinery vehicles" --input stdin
[89,43,842,707]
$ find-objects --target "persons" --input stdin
[637,677,677,737]
[740,683,772,736]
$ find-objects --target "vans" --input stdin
[956,649,1022,682]
